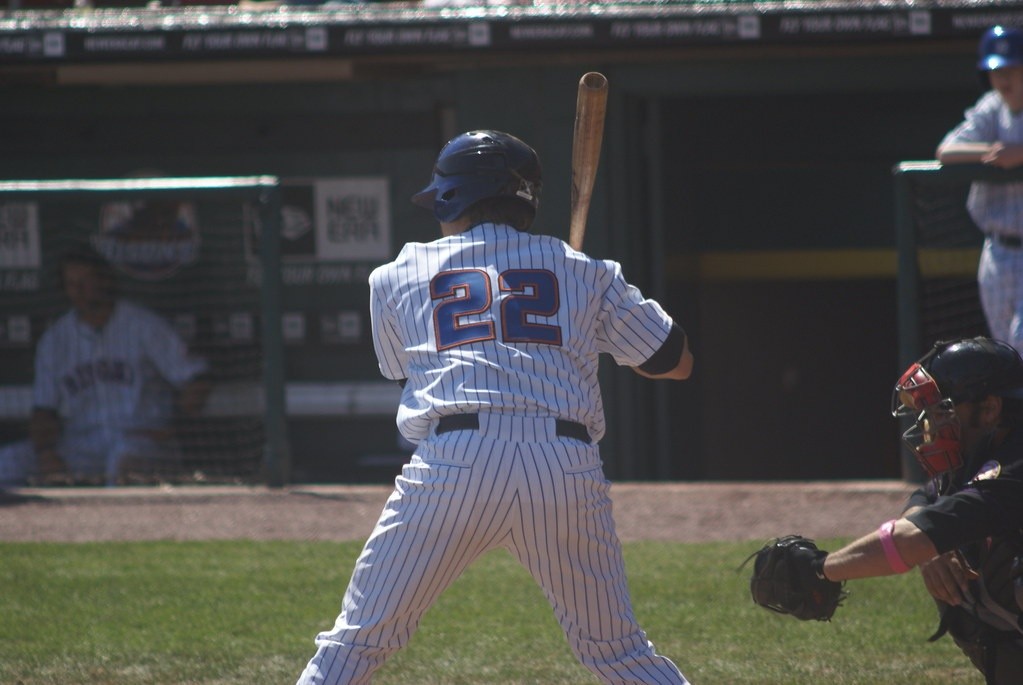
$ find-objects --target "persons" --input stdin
[937,25,1023,357]
[298,130,696,685]
[751,333,1023,683]
[31,247,215,478]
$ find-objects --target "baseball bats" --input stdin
[567,70,610,252]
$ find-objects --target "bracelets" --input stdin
[880,519,910,575]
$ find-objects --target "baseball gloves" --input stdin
[749,536,843,623]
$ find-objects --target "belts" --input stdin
[986,231,1023,250]
[435,413,592,445]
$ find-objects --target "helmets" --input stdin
[921,339,1023,400]
[411,130,540,225]
[979,25,1023,71]
